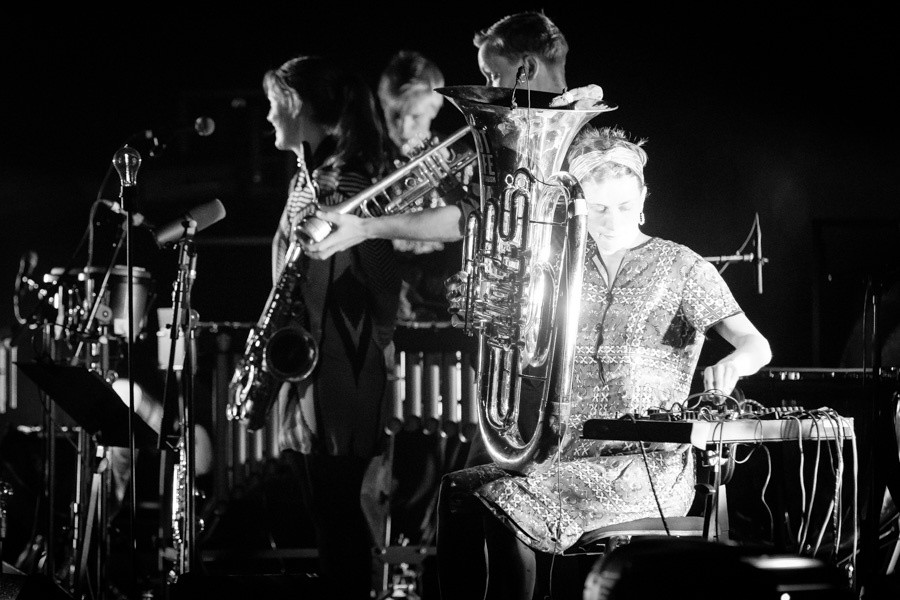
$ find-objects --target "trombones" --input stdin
[294,122,477,253]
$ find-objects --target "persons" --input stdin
[260,57,396,589]
[300,12,570,261]
[370,51,480,350]
[436,125,773,599]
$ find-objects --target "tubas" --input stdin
[430,83,619,476]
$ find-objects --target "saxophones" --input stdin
[225,238,320,430]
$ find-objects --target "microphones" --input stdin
[150,198,227,246]
